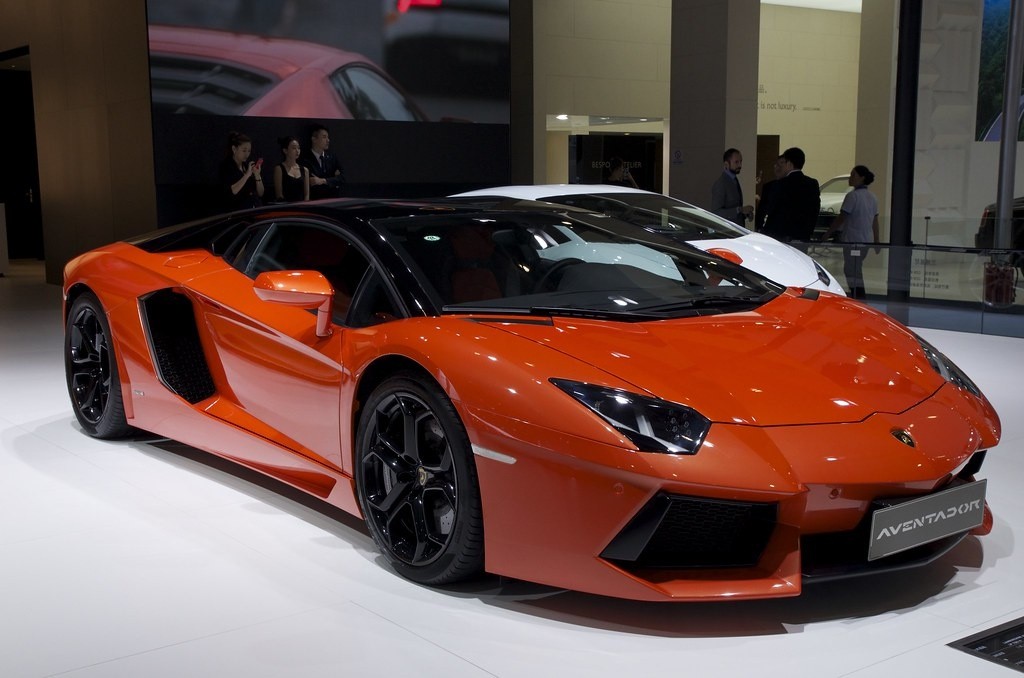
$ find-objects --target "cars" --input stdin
[807,174,857,245]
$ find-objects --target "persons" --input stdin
[755,147,822,243]
[755,154,792,235]
[214,131,272,211]
[303,129,341,201]
[600,158,640,189]
[710,147,754,231]
[820,165,882,300]
[273,138,310,203]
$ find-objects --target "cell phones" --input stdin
[254,158,263,170]
[623,168,629,180]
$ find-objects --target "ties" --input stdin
[734,177,743,204]
[320,156,325,174]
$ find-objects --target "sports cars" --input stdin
[56,191,1002,605]
[445,185,847,311]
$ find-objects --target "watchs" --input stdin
[255,177,261,182]
